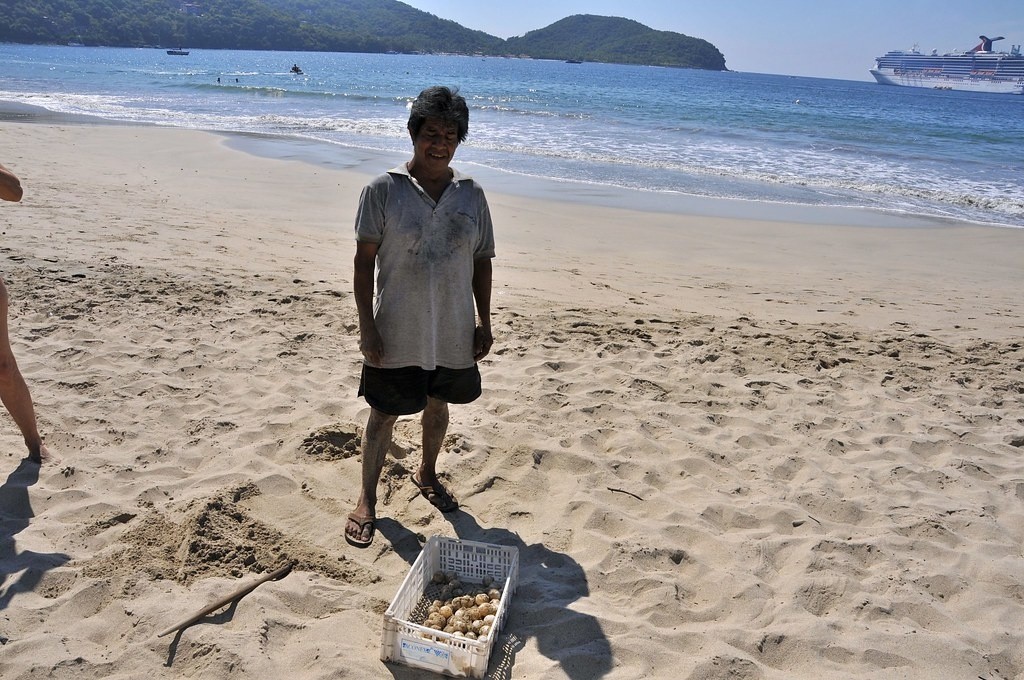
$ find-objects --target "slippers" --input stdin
[344,470,459,545]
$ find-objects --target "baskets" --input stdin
[381,534,519,679]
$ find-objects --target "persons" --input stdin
[0,164,60,468]
[343,86,495,545]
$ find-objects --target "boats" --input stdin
[166,50,190,56]
[291,64,303,74]
[566,58,582,64]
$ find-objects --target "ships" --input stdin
[869,36,1024,96]
